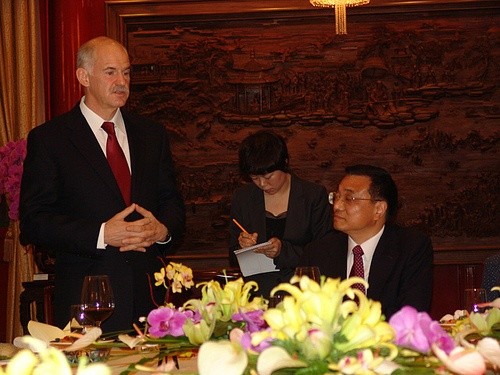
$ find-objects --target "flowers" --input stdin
[0,138,27,220]
[5,262,500,375]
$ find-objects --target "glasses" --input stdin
[328,192,383,206]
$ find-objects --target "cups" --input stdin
[262,294,282,309]
[293,266,320,287]
[70,304,94,336]
[463,288,487,315]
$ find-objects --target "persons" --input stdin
[20,36,187,339]
[228,131,337,302]
[304,165,434,323]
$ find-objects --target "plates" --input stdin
[49,339,115,346]
[439,323,456,327]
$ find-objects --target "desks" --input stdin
[20,280,54,335]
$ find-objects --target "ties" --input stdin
[101,122,131,208]
[342,245,365,314]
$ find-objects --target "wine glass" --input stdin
[80,275,114,341]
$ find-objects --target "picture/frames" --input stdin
[104,0,500,270]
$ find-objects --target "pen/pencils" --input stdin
[232,219,256,244]
